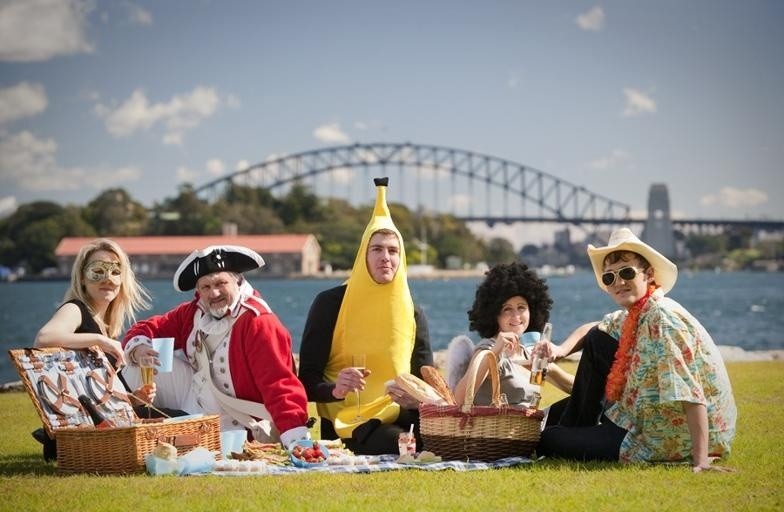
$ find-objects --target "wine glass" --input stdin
[351,354,367,419]
[139,355,155,419]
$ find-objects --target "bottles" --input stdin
[529,323,555,387]
[77,393,117,428]
[500,390,543,409]
[397,431,417,460]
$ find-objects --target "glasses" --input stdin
[601,265,646,284]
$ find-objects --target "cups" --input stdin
[519,331,542,357]
[151,336,174,374]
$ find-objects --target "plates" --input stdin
[38,372,80,416]
[87,367,127,407]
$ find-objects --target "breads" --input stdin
[396,372,449,407]
[386,380,421,411]
[421,365,458,408]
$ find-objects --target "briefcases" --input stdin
[9,343,223,475]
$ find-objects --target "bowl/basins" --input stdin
[288,440,330,468]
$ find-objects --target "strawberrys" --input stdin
[292,441,326,464]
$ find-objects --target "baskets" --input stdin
[418,350,544,463]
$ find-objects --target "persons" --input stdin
[299,231,435,454]
[33,239,153,463]
[441,262,556,407]
[530,228,738,473]
[120,245,316,451]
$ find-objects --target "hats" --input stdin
[173,244,266,293]
[586,227,679,295]
